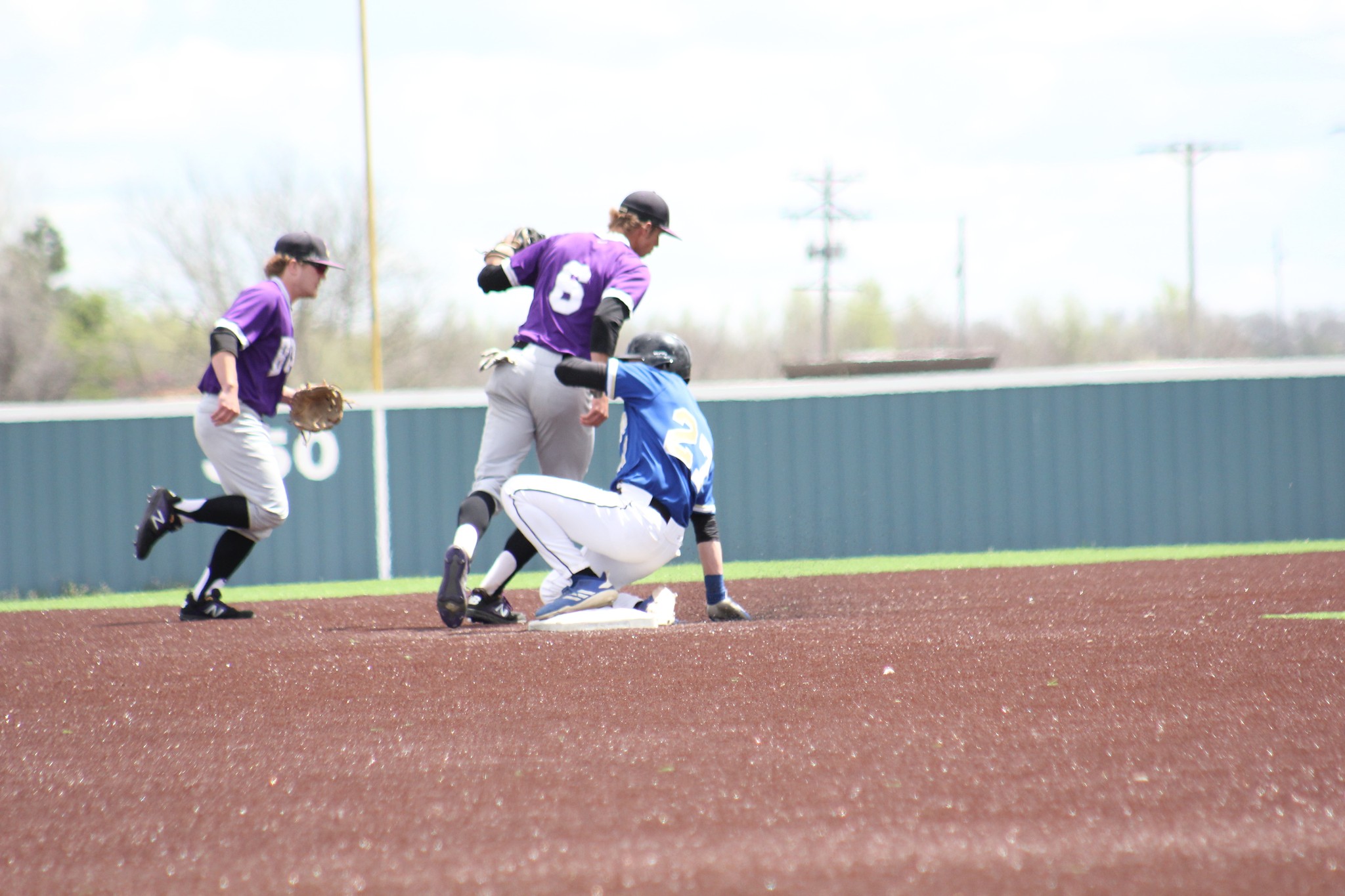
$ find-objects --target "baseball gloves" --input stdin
[484,226,546,260]
[290,385,343,432]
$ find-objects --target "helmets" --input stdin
[616,333,691,380]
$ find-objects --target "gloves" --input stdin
[706,596,751,622]
[478,347,514,371]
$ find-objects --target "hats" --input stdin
[274,231,345,270]
[619,190,682,241]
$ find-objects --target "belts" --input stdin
[615,488,671,524]
[511,340,572,361]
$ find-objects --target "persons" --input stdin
[134,232,346,622]
[500,334,752,626]
[437,192,679,630]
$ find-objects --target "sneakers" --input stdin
[535,572,618,621]
[638,587,663,612]
[180,589,254,622]
[437,546,471,629]
[132,485,183,561]
[465,588,527,624]
